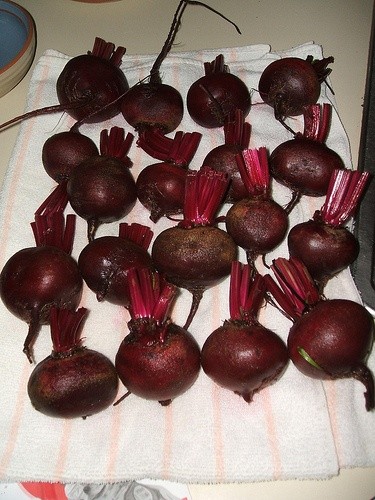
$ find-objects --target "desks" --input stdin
[0,0,375,500]
